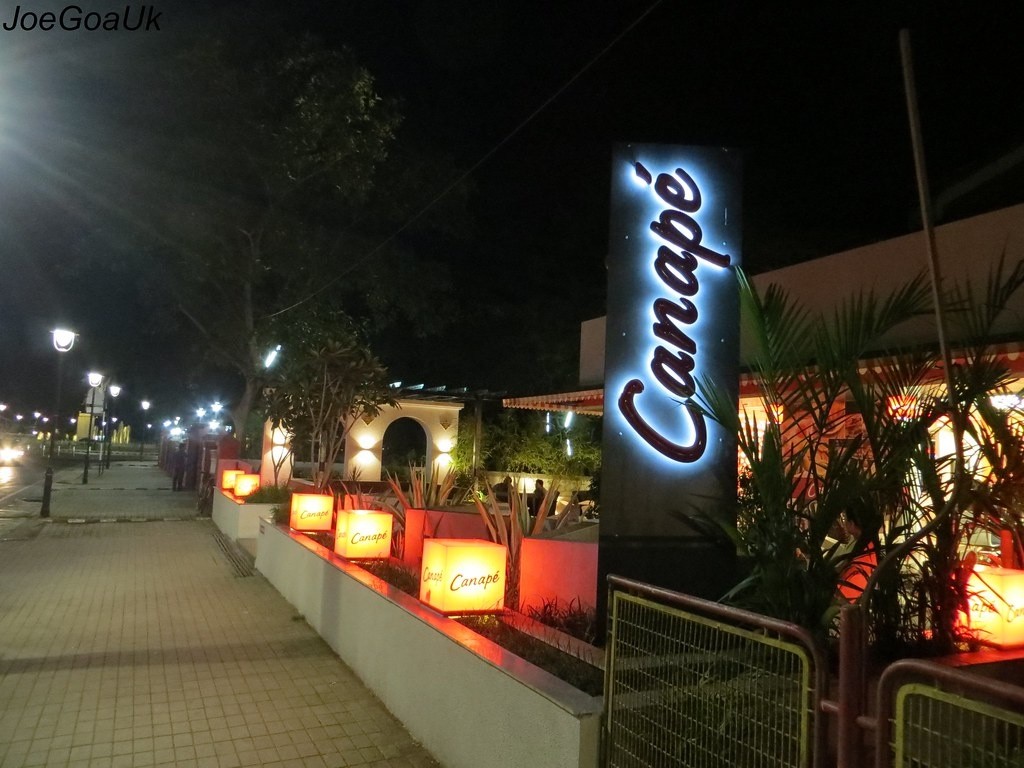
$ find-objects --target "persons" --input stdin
[832,501,878,605]
[171,443,190,491]
[492,477,513,502]
[532,479,548,517]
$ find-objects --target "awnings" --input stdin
[502,341,1024,416]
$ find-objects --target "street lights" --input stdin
[39,325,82,519]
[105,385,122,470]
[138,399,151,461]
[82,370,105,485]
